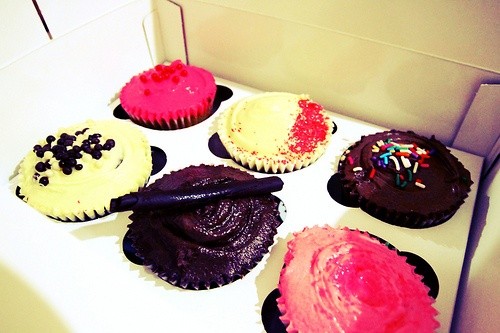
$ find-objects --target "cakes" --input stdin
[215,92,335,173]
[339,129,474,230]
[119,58,216,130]
[277,224,441,333]
[110,164,284,287]
[19,118,152,222]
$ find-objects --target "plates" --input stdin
[207,121,338,174]
[122,194,286,291]
[15,145,168,222]
[261,230,439,332]
[113,85,234,131]
[327,145,470,229]
